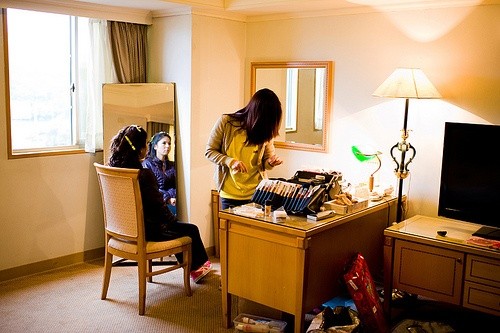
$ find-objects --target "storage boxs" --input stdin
[232,312,288,333]
[324,197,368,217]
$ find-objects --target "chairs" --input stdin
[93,161,194,316]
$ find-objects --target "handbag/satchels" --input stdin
[343,252,388,333]
[253,168,343,214]
[305,305,361,333]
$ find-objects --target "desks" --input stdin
[382,215,500,320]
[218,192,407,333]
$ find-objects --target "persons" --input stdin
[104,124,213,284]
[142,131,176,216]
[205,88,284,290]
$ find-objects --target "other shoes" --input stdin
[190,260,213,283]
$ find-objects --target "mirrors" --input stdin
[102,83,176,266]
[248,60,335,153]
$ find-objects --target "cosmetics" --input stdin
[275,211,286,220]
[264,200,272,216]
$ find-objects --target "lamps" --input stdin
[352,146,385,202]
[371,66,444,332]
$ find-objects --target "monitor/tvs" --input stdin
[437,121,500,242]
